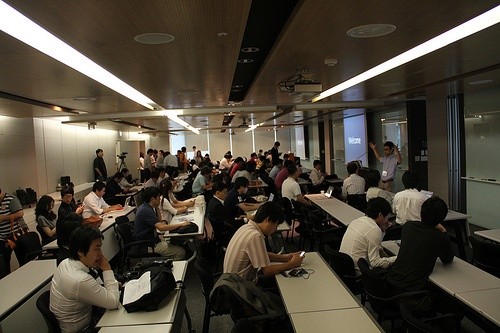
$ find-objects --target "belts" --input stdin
[382,179,394,183]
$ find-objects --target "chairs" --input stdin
[8,160,500,333]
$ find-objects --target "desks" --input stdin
[289,304,386,333]
[44,205,137,266]
[454,287,500,326]
[97,323,174,333]
[305,195,365,229]
[270,251,363,316]
[96,260,189,327]
[163,195,205,237]
[380,241,500,297]
[1,260,59,333]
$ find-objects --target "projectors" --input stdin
[279,83,322,95]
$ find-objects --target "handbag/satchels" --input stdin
[6,214,28,249]
[169,222,199,234]
[83,216,103,229]
[119,265,176,313]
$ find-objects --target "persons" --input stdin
[0,188,31,281]
[340,162,366,201]
[381,195,458,331]
[49,224,121,333]
[92,148,107,181]
[34,141,434,262]
[223,202,307,293]
[81,181,123,221]
[338,197,399,307]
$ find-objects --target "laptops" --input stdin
[312,186,334,200]
[268,193,274,202]
[110,196,131,211]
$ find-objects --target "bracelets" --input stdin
[374,151,377,153]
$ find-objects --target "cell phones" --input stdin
[300,251,305,257]
